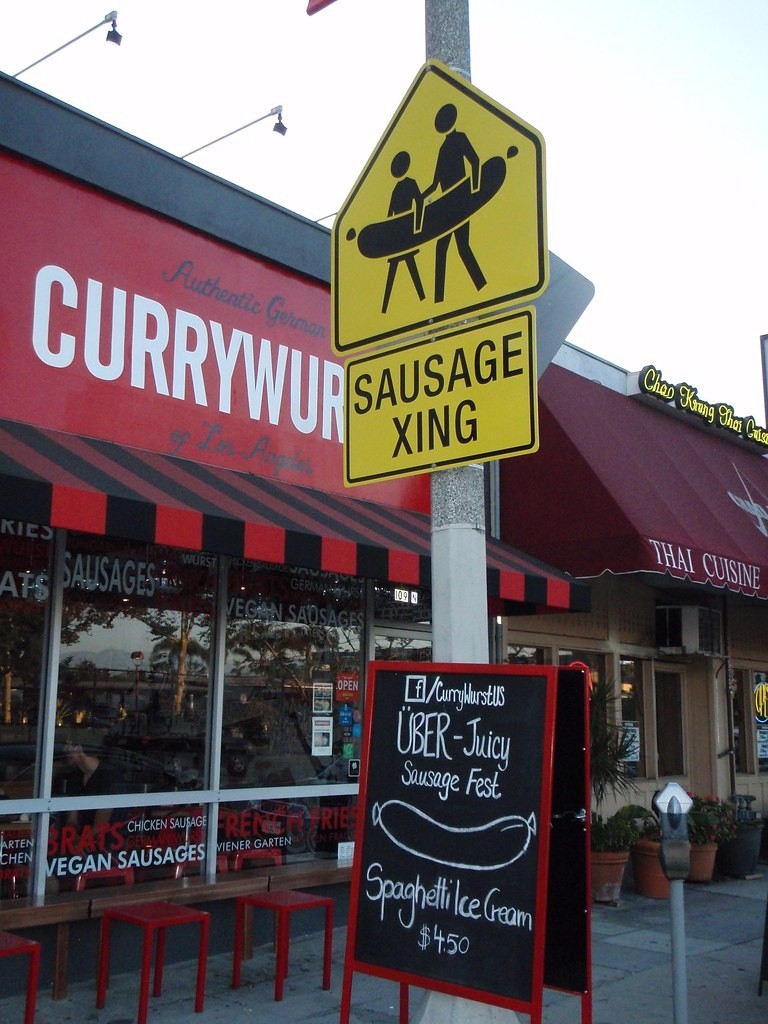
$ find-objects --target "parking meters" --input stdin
[652,782,698,1023]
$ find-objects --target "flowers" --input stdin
[686,791,734,846]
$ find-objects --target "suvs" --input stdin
[194,710,293,777]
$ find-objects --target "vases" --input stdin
[688,843,717,882]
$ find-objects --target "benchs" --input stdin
[0,853,354,999]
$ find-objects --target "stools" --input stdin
[1,889,334,1024]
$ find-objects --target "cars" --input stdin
[0,742,201,898]
[243,753,362,870]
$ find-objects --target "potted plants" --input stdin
[591,792,676,905]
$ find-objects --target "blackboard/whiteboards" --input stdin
[351,661,593,1015]
